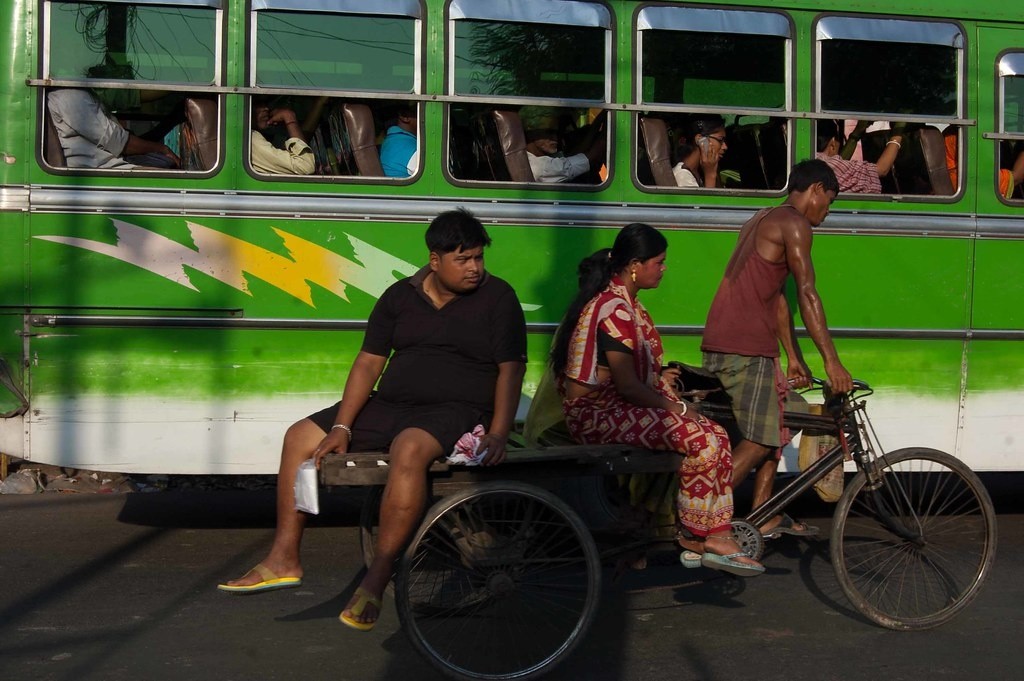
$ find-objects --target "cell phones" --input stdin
[697,136,710,157]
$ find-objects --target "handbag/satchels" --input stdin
[798,380,844,503]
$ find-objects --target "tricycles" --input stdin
[319,377,998,681]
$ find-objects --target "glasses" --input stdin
[700,131,727,145]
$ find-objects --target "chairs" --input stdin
[44,93,956,196]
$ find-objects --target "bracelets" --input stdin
[885,141,901,149]
[331,424,352,442]
[675,400,687,416]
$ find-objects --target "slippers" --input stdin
[762,512,820,537]
[217,564,302,595]
[339,586,384,632]
[702,551,766,577]
[680,550,703,569]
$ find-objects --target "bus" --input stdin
[0,0,1024,511]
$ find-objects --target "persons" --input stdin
[44,74,189,170]
[515,100,609,185]
[637,109,730,188]
[941,119,1024,203]
[251,80,337,178]
[381,93,423,180]
[524,224,768,575]
[697,158,855,534]
[817,118,911,195]
[217,205,527,627]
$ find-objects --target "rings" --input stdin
[316,448,320,451]
